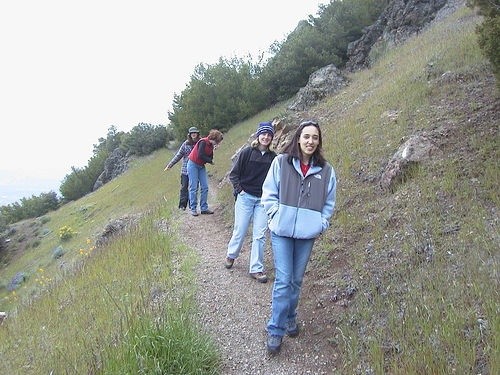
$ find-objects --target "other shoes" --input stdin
[201,209,214,214]
[180,207,185,212]
[191,211,198,215]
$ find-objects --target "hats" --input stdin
[257,122,275,137]
[188,127,199,133]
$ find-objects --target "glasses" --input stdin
[298,121,317,129]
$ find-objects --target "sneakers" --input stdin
[250,272,267,282]
[267,335,282,352]
[225,256,234,268]
[286,321,298,336]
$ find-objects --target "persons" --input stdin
[164,127,202,210]
[225,123,277,283]
[260,121,337,352]
[187,130,224,216]
